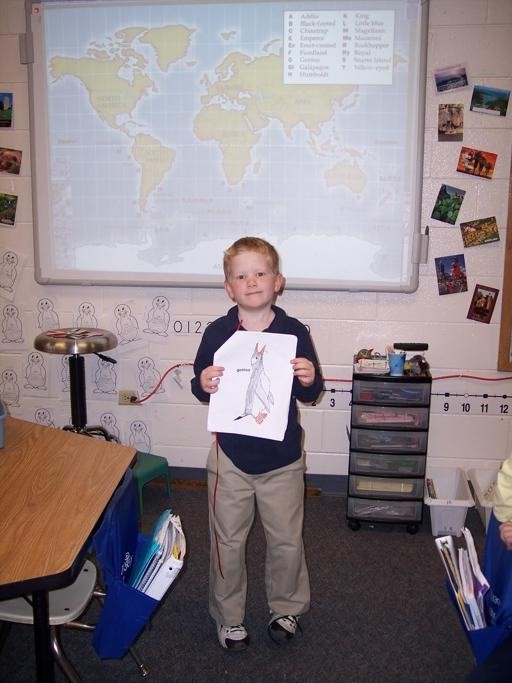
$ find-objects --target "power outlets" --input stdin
[118,390,136,405]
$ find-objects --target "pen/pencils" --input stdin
[386,344,394,354]
[425,225,430,236]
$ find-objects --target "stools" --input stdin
[135,451,174,521]
[32,325,118,445]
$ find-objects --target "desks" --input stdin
[1,419,137,683]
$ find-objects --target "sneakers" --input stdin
[215,622,250,653]
[267,609,299,645]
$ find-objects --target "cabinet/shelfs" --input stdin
[343,371,433,536]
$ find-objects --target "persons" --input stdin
[191,237,324,653]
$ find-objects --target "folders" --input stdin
[146,514,187,601]
[436,534,476,633]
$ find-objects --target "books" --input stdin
[130,508,187,603]
[434,526,491,632]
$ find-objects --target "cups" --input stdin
[387,351,405,376]
